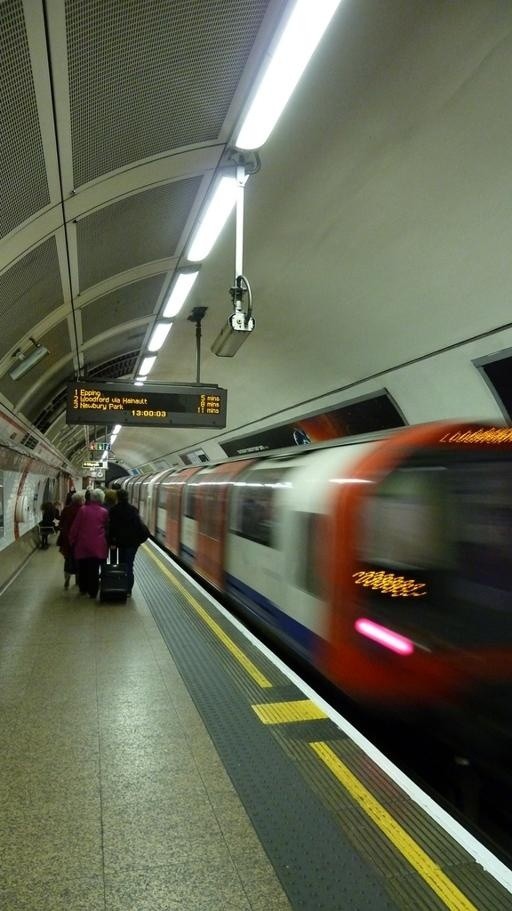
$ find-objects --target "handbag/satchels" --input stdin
[139,524,150,544]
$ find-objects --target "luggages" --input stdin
[100,547,128,603]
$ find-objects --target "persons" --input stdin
[38,482,143,599]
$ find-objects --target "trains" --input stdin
[109,419,512,710]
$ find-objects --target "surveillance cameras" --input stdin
[210,311,254,357]
